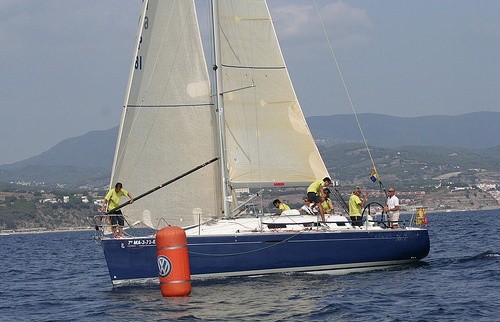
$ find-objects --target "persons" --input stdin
[349,189,366,226]
[298,188,334,215]
[307,177,331,220]
[384,187,399,226]
[100,183,133,238]
[273,199,290,213]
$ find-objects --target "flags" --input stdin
[370,173,378,182]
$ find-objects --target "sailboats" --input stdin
[89,0,431,284]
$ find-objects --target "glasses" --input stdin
[388,191,393,192]
[357,191,361,193]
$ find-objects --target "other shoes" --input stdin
[320,223,330,229]
